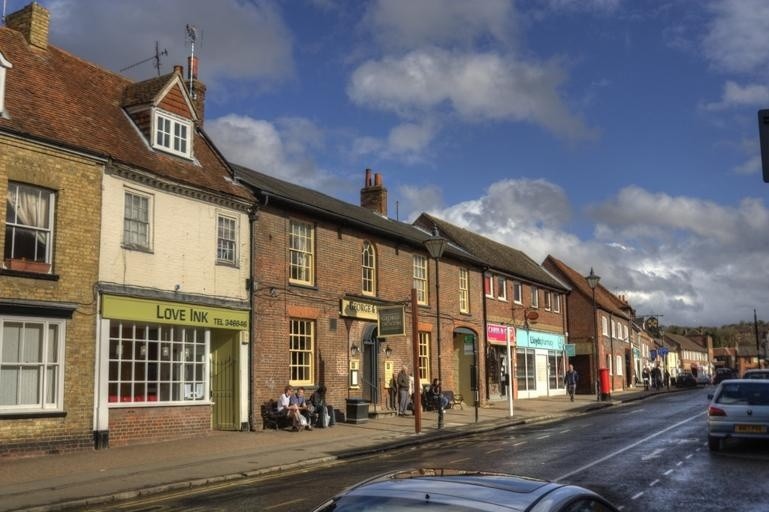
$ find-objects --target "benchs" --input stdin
[412,390,453,411]
[262,399,333,430]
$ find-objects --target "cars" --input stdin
[307,468,624,512]
[674,372,695,388]
[712,367,734,386]
[705,379,769,443]
[737,368,769,382]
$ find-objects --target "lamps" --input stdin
[116,328,190,357]
[385,345,392,358]
[351,343,359,356]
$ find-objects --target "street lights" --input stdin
[658,325,670,390]
[584,263,601,401]
[419,219,449,429]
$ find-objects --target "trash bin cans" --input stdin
[345,398,372,424]
[671,378,675,387]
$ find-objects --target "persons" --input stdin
[277,385,334,431]
[388,364,449,417]
[562,364,580,403]
[642,363,663,392]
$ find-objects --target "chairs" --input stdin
[452,394,464,410]
